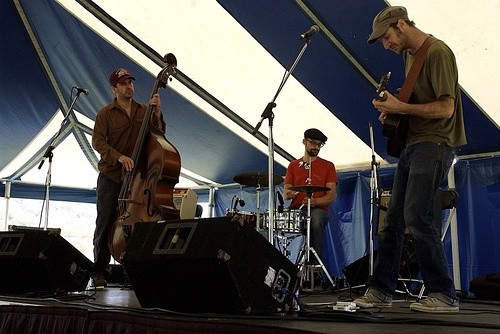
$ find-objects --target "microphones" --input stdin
[278,191,284,206]
[235,196,245,207]
[298,25,320,40]
[73,86,90,95]
[373,203,387,211]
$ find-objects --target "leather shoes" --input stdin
[89,275,107,287]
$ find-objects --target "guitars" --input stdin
[376,72,409,158]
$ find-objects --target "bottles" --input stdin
[292,289,300,311]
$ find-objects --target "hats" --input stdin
[304,129,327,144]
[367,6,408,44]
[110,68,136,86]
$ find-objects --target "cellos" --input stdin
[109,53,181,290]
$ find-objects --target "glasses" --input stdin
[306,139,324,148]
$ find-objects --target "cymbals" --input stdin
[288,185,331,192]
[233,172,284,187]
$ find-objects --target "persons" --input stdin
[353,7,467,313]
[89,68,167,287]
[282,129,338,286]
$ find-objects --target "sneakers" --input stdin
[410,297,460,313]
[354,288,393,307]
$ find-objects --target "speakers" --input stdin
[122,214,301,317]
[0,230,95,296]
[172,188,198,220]
[376,188,412,236]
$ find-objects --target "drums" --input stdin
[225,209,263,229]
[264,208,307,233]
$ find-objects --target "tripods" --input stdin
[296,192,339,295]
[340,121,408,299]
